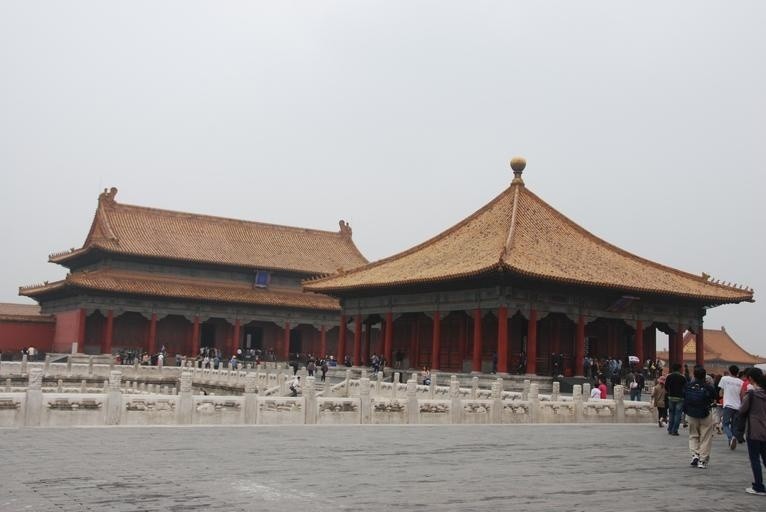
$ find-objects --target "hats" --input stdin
[657,376,666,384]
[694,368,706,377]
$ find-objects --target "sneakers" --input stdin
[730,436,737,450]
[690,455,707,468]
[745,488,766,496]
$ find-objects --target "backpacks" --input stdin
[684,383,709,417]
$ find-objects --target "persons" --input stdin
[394,348,404,369]
[516,351,649,401]
[289,376,301,397]
[649,358,766,497]
[27,344,37,362]
[114,342,387,381]
[21,346,28,355]
[199,387,207,395]
[421,365,430,384]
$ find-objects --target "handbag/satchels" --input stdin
[630,379,638,389]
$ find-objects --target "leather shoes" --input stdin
[658,420,664,427]
[669,432,679,435]
[683,425,689,428]
